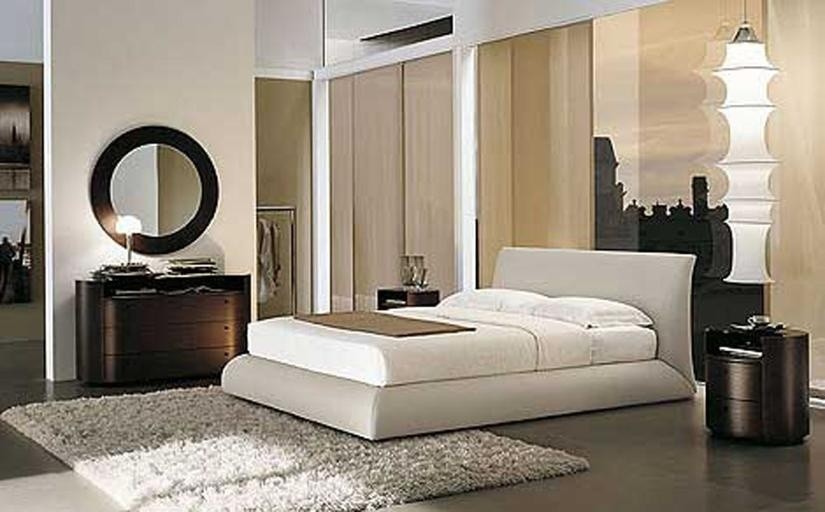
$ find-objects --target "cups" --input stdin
[748,315,769,327]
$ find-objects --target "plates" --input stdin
[731,323,789,331]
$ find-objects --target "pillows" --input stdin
[532,295,654,330]
[436,286,551,318]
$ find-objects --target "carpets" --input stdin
[1,384,592,511]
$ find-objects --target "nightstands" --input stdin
[375,287,440,311]
[702,326,810,447]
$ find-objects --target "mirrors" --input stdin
[89,125,219,255]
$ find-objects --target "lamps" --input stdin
[114,214,144,277]
[710,0,781,286]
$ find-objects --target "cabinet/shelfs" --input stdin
[75,272,251,388]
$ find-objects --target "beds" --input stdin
[220,246,699,441]
